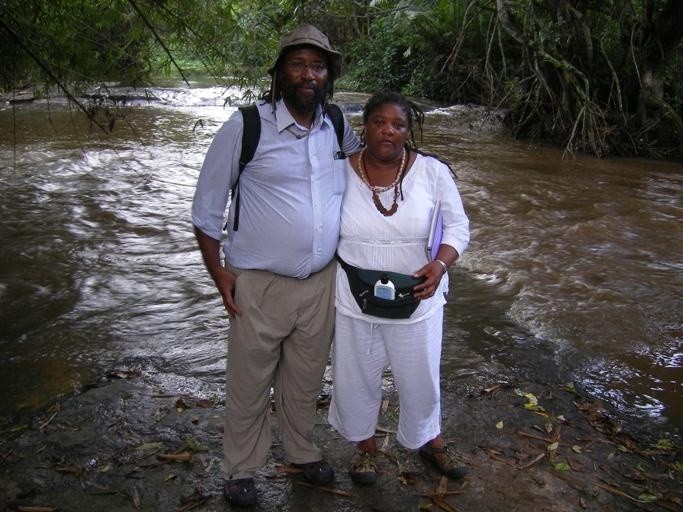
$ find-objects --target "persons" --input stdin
[188,24,366,510]
[325,90,472,487]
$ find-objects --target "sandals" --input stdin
[419,443,470,478]
[350,447,378,484]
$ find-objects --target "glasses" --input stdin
[281,58,329,74]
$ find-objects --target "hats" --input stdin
[267,24,343,82]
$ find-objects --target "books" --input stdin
[424,200,450,307]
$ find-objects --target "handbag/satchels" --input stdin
[334,253,427,319]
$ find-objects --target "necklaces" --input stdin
[356,144,408,218]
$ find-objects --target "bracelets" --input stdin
[434,259,448,275]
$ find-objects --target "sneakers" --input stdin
[291,456,335,486]
[224,476,257,509]
